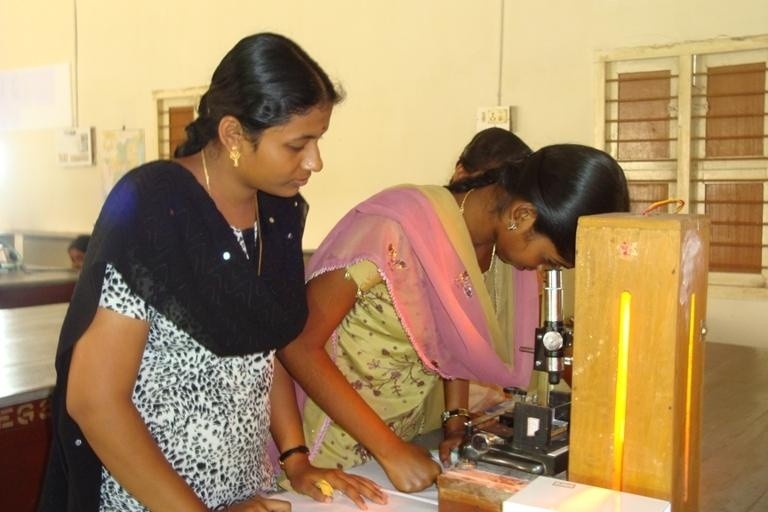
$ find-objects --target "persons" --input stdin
[67,235,90,265]
[267,144,632,493]
[442,125,549,429]
[43,32,388,512]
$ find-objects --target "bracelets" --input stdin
[275,444,309,470]
[439,408,470,424]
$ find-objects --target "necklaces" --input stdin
[458,187,497,274]
[199,148,263,278]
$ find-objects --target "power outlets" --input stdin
[486,107,509,124]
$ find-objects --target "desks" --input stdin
[267,343,767,511]
